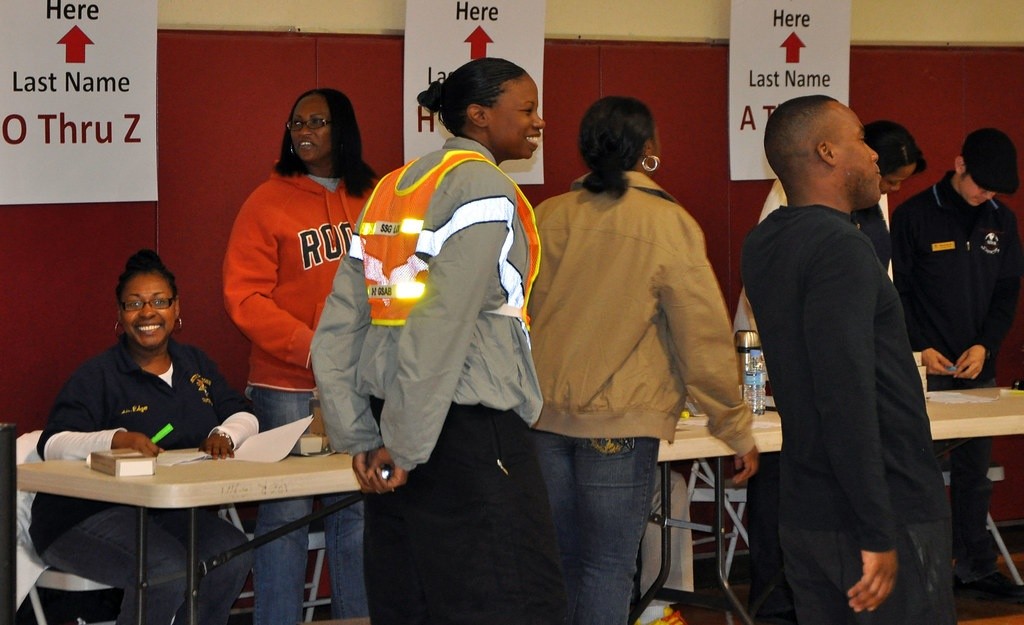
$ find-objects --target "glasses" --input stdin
[286,117,333,132]
[120,295,175,311]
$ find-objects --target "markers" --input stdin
[151,423,174,443]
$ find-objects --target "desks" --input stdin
[18,387,1024,625]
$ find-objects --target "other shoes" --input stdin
[634,604,688,625]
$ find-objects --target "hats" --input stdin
[961,128,1020,194]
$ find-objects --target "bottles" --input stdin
[744,350,766,416]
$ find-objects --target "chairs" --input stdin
[216,502,325,625]
[17,431,124,625]
[682,458,751,582]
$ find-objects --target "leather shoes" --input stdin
[952,573,1024,603]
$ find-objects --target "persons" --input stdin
[889,128,1024,604]
[224,85,380,625]
[729,120,928,383]
[739,94,958,625]
[27,250,259,625]
[309,58,546,625]
[523,94,761,625]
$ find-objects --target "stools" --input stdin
[939,464,1023,589]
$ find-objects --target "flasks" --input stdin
[734,330,761,400]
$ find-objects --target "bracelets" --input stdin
[219,433,232,445]
